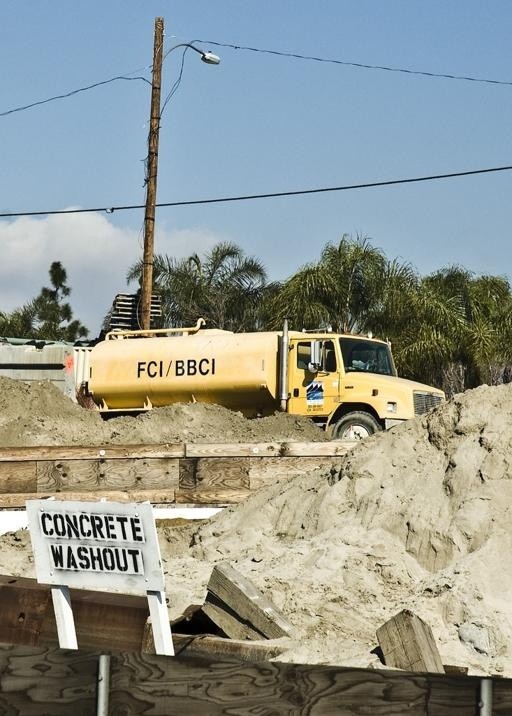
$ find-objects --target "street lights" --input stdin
[134,43,221,330]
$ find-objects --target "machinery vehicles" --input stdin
[82,316,447,441]
